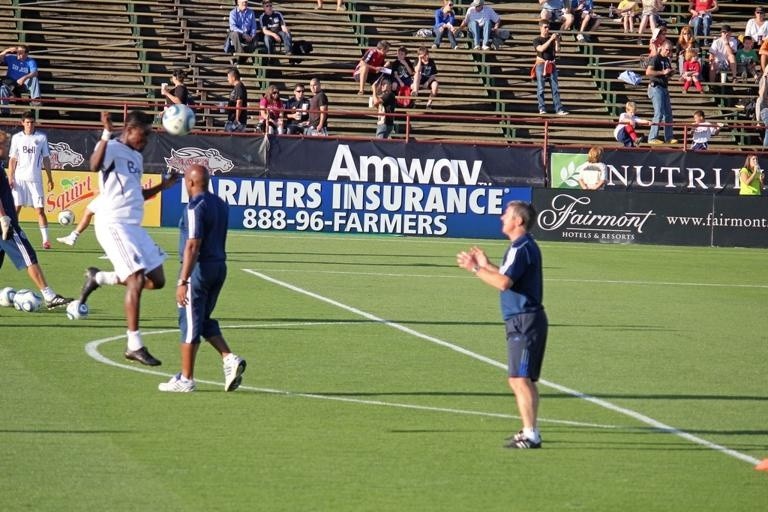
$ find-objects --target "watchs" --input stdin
[471,265,481,275]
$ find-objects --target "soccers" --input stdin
[22,291,42,312]
[162,104,196,136]
[13,289,31,311]
[0,286,15,307]
[66,299,88,321]
[58,209,75,226]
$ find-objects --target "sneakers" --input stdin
[474,45,480,50]
[648,139,664,145]
[56,235,77,248]
[504,430,542,441]
[45,294,74,310]
[634,136,643,147]
[79,266,102,305]
[222,356,247,392]
[125,346,162,366]
[482,45,490,51]
[43,241,51,249]
[556,111,569,116]
[539,110,547,114]
[665,138,678,144]
[504,438,542,449]
[158,373,196,392]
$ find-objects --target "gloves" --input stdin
[0,216,14,241]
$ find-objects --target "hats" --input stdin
[471,0,485,7]
[720,24,731,34]
[756,5,765,13]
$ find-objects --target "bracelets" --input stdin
[98,129,114,142]
[177,278,189,286]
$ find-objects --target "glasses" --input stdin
[296,90,304,93]
[272,92,279,95]
[449,4,453,6]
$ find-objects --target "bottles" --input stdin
[609,3,614,17]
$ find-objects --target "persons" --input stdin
[226,67,329,137]
[0,130,74,310]
[158,164,246,393]
[355,41,437,135]
[8,111,54,249]
[228,0,259,64]
[259,0,295,65]
[56,193,110,259]
[533,1,768,149]
[457,200,548,449]
[80,110,183,367]
[0,45,41,116]
[740,155,765,196]
[156,69,188,118]
[458,0,500,51]
[431,0,460,49]
[578,145,608,190]
[314,0,346,11]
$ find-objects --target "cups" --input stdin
[302,104,309,115]
[296,109,301,120]
[219,101,225,113]
[720,73,727,83]
[369,96,374,108]
[161,83,168,95]
